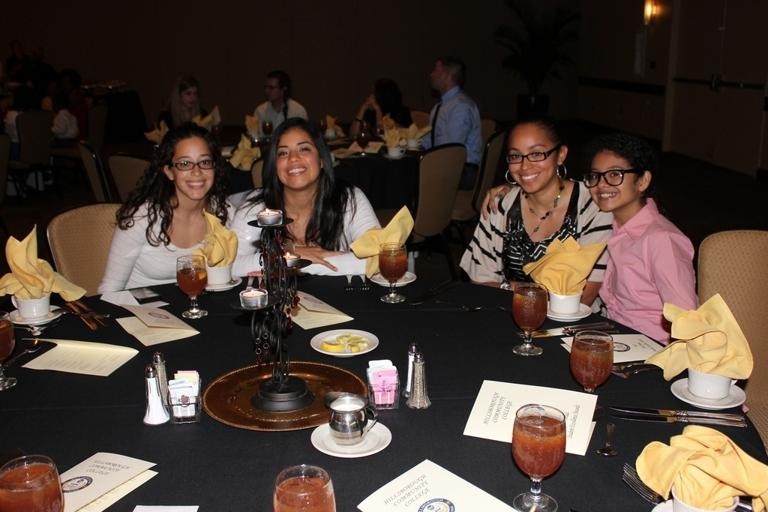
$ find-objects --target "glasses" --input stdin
[505,142,562,164]
[582,166,643,189]
[168,158,218,171]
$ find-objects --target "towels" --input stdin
[635,426,768,512]
[0,224,86,301]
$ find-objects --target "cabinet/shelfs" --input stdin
[672,1,768,180]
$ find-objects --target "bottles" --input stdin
[143,353,172,425]
[402,342,431,410]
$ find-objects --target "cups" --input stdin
[256,210,282,226]
[283,254,301,267]
[12,292,52,318]
[322,120,421,159]
[687,366,738,400]
[0,454,64,512]
[326,391,379,446]
[671,483,753,512]
[240,287,268,310]
[273,465,336,512]
[205,262,232,285]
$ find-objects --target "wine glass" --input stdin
[176,255,208,319]
[251,120,273,143]
[509,281,614,512]
[379,242,408,303]
[0,312,17,391]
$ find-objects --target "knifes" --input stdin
[66,299,108,330]
[607,402,747,428]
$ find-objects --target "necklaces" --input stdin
[521,175,565,237]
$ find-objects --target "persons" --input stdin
[251,72,307,143]
[155,73,221,140]
[349,79,417,139]
[3,86,104,192]
[97,121,244,294]
[396,55,483,248]
[227,118,391,277]
[479,135,699,346]
[459,113,615,309]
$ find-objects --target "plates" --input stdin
[202,361,369,432]
[309,329,379,357]
[5,305,62,325]
[204,275,242,291]
[671,378,746,411]
[651,499,737,512]
[311,420,392,458]
[371,272,417,287]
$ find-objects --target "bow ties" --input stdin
[430,101,442,149]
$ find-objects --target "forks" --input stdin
[345,274,370,292]
[610,360,659,379]
[622,465,663,505]
[9,343,41,366]
